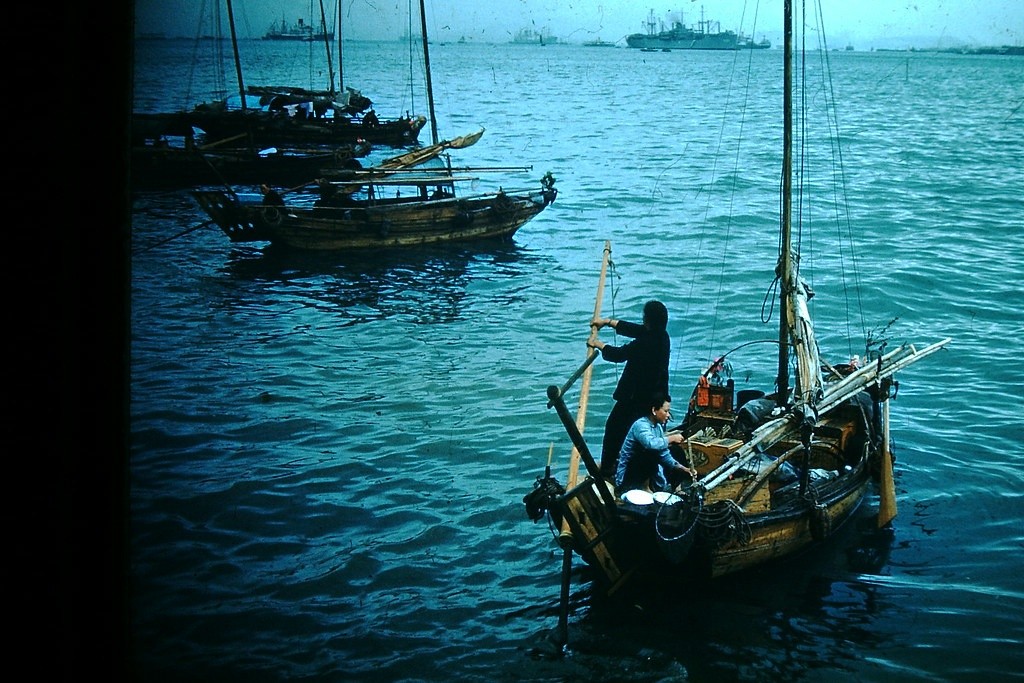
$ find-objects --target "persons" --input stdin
[362,110,380,125]
[258,181,282,205]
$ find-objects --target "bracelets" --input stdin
[583,302,698,493]
[608,319,613,324]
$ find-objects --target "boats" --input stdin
[662,48,672,52]
[262,19,335,41]
[585,41,615,47]
[641,47,658,52]
[624,6,772,52]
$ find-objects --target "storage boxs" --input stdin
[683,387,864,511]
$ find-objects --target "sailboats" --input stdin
[533,0,953,627]
[131,0,556,253]
